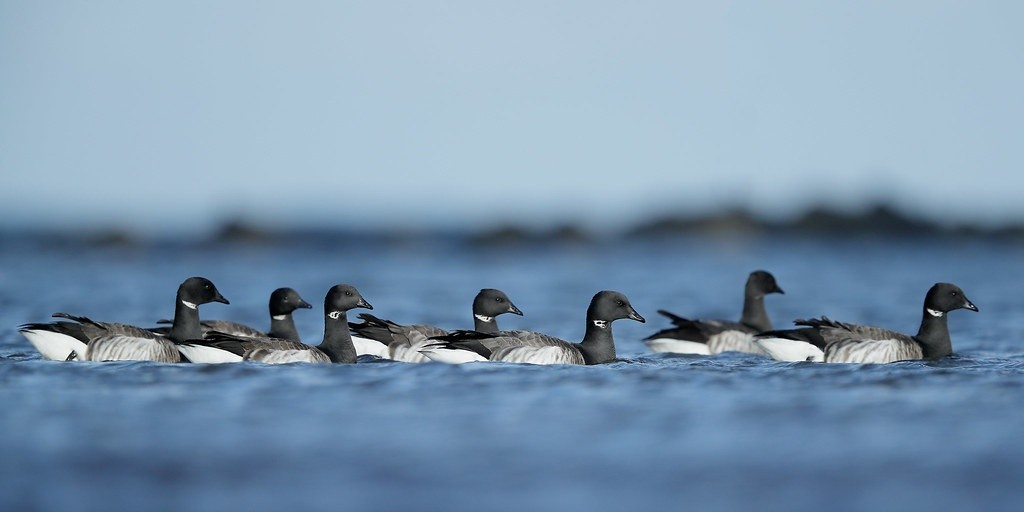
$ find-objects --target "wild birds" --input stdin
[347,288,525,366]
[142,288,313,343]
[174,284,374,365]
[418,291,646,366]
[642,270,786,353]
[754,283,979,361]
[17,277,231,364]
